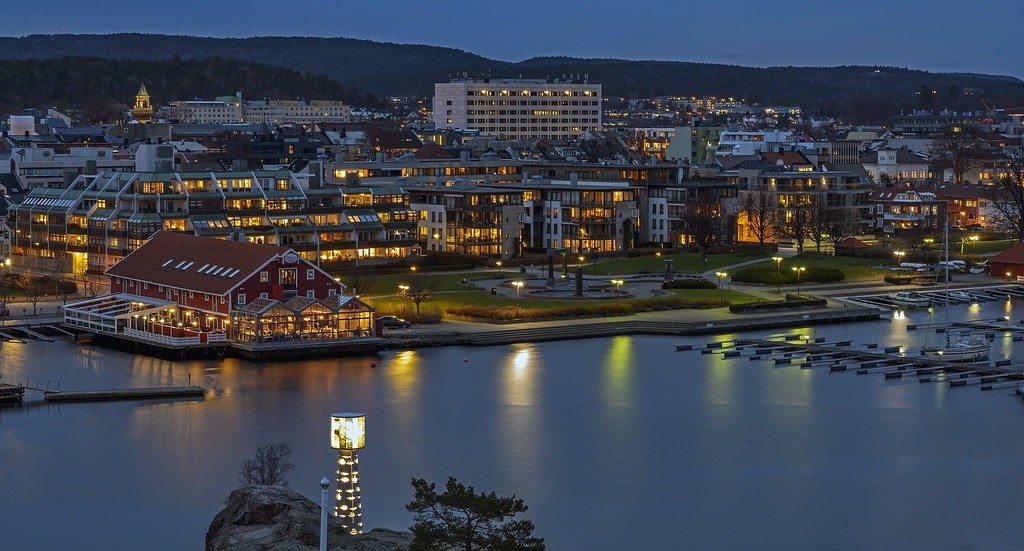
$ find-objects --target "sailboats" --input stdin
[920,218,990,361]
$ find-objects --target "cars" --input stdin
[378,315,411,330]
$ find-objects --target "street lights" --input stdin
[512,281,523,320]
[715,272,728,303]
[792,267,806,296]
[924,239,933,265]
[611,279,624,317]
[398,285,410,312]
[893,251,906,277]
[772,257,783,294]
